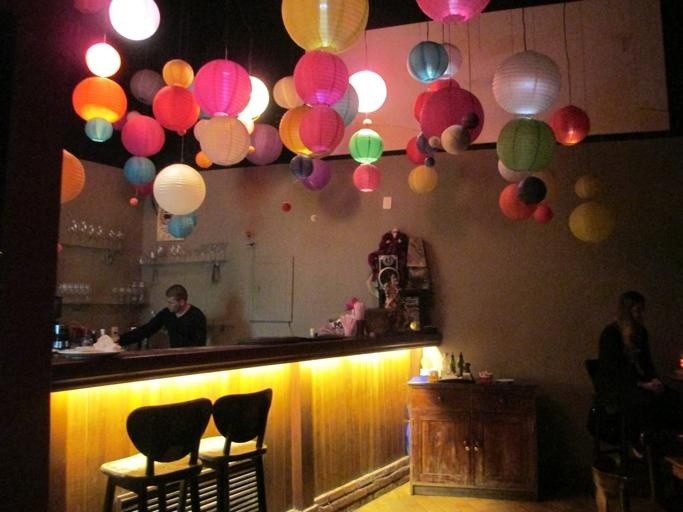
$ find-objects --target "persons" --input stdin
[112,283,208,350]
[597,291,683,461]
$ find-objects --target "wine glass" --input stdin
[110,281,147,307]
[136,240,227,266]
[64,218,123,252]
[56,283,93,303]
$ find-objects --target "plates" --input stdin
[496,378,514,383]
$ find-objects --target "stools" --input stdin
[585,455,647,512]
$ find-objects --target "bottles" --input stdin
[449,352,455,373]
[463,363,472,381]
[429,370,438,381]
[456,351,464,377]
[441,353,448,371]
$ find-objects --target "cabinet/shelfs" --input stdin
[55,239,147,313]
[409,378,540,501]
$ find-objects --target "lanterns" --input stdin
[58,2,618,248]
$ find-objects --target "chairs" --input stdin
[194,386,270,510]
[98,397,213,511]
[585,357,663,507]
